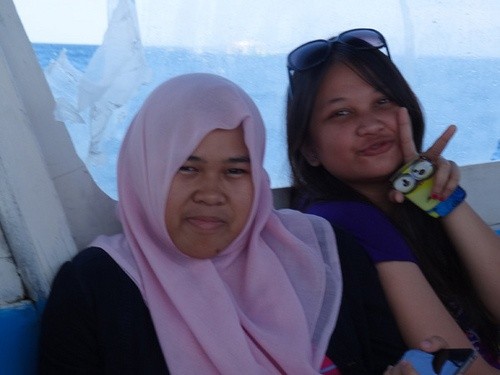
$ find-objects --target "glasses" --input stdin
[287,29,391,100]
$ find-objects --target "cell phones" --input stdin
[401,348,476,375]
[391,156,467,218]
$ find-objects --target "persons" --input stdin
[283,38,500,374]
[35,70,420,375]
